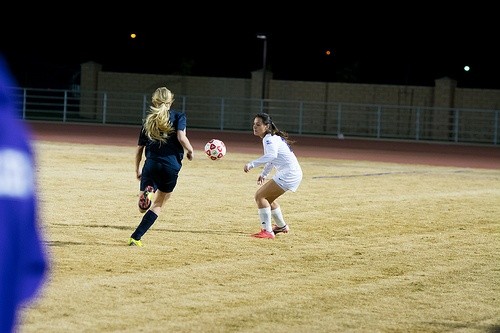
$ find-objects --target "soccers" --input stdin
[204,139,226,161]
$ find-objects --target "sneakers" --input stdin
[272,224,289,234]
[139,185,154,210]
[128,238,143,247]
[251,228,276,240]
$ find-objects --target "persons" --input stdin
[244,112,303,240]
[128,87,195,248]
[0,71,51,332]
